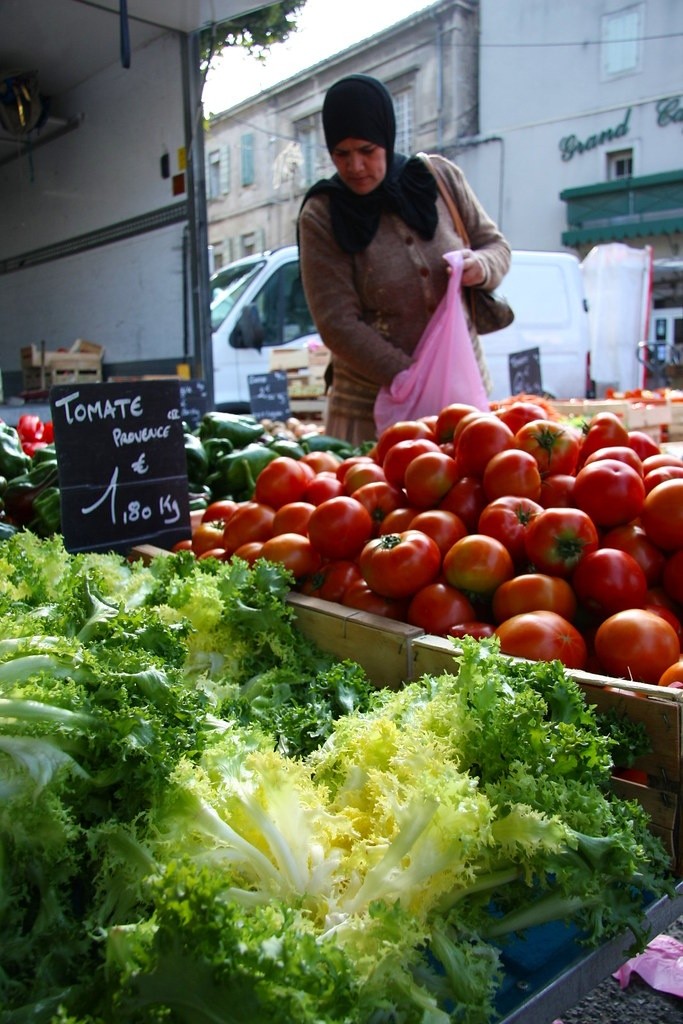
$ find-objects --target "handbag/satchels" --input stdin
[416,152,514,334]
[373,252,488,438]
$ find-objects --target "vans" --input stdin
[210,243,653,415]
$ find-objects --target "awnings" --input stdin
[561,216,683,259]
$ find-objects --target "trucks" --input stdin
[0,0,288,427]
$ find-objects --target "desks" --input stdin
[487,877,683,1024]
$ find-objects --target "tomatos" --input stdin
[176,401,683,788]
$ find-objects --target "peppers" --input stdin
[0,410,378,543]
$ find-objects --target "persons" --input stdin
[296,74,511,448]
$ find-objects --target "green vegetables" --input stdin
[0,525,679,1024]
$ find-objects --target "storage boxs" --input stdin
[21,337,105,369]
[547,398,683,461]
[611,773,683,872]
[131,540,420,692]
[266,343,331,426]
[22,368,103,392]
[403,631,683,777]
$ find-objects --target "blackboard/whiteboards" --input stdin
[49,378,198,559]
[177,379,208,431]
[248,371,291,423]
[508,347,542,397]
